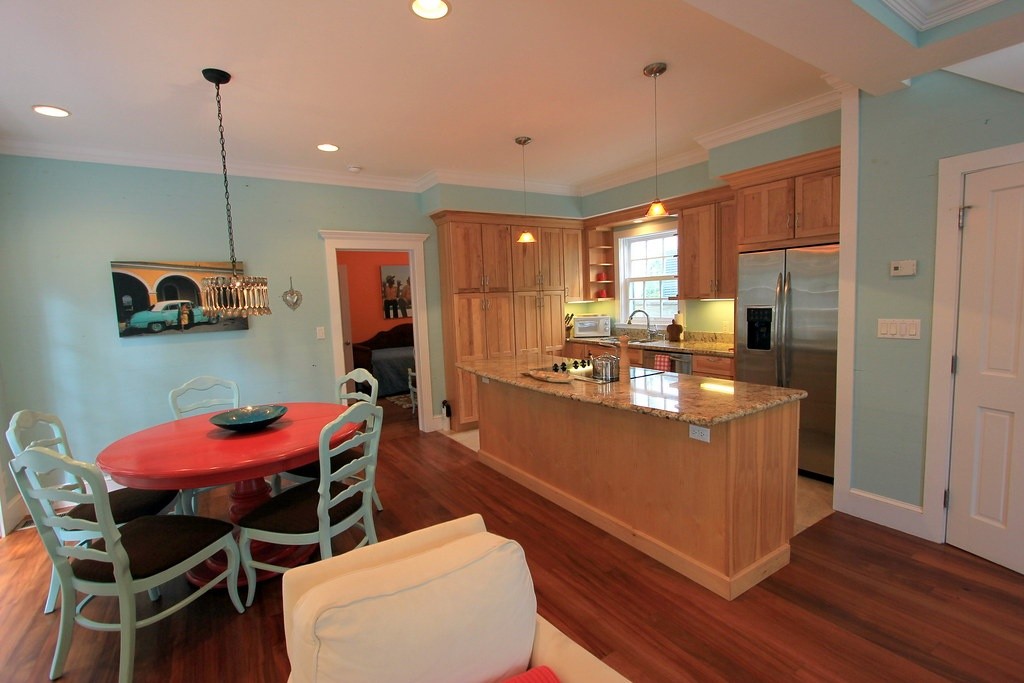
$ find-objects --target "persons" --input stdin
[180,304,189,332]
[382,275,412,318]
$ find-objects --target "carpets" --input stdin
[385,393,416,410]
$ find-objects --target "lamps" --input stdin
[200,69,272,317]
[411,0,451,22]
[516,137,537,245]
[644,63,672,219]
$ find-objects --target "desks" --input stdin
[95,402,363,590]
[455,350,808,602]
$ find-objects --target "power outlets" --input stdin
[689,425,711,443]
[890,260,917,277]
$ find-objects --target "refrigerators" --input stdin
[733,242,840,485]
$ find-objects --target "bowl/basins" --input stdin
[207,404,289,432]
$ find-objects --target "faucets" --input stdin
[658,333,667,340]
[626,309,659,340]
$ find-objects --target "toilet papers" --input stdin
[674,313,684,340]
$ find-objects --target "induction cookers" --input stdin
[529,357,665,384]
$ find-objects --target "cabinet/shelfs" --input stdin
[436,220,585,432]
[672,166,841,302]
[586,225,617,301]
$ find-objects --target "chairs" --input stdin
[407,368,417,413]
[7,447,245,682]
[230,402,383,606]
[335,367,384,512]
[169,374,240,517]
[5,408,160,615]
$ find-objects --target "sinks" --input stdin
[601,338,638,342]
[628,340,654,344]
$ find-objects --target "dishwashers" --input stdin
[642,351,692,375]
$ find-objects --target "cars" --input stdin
[128,299,219,333]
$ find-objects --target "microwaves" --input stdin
[572,315,611,337]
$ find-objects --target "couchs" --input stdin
[352,323,416,401]
[278,511,631,683]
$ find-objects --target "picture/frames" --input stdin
[379,264,412,320]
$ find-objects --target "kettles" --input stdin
[588,352,620,382]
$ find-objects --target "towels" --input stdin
[653,353,671,372]
